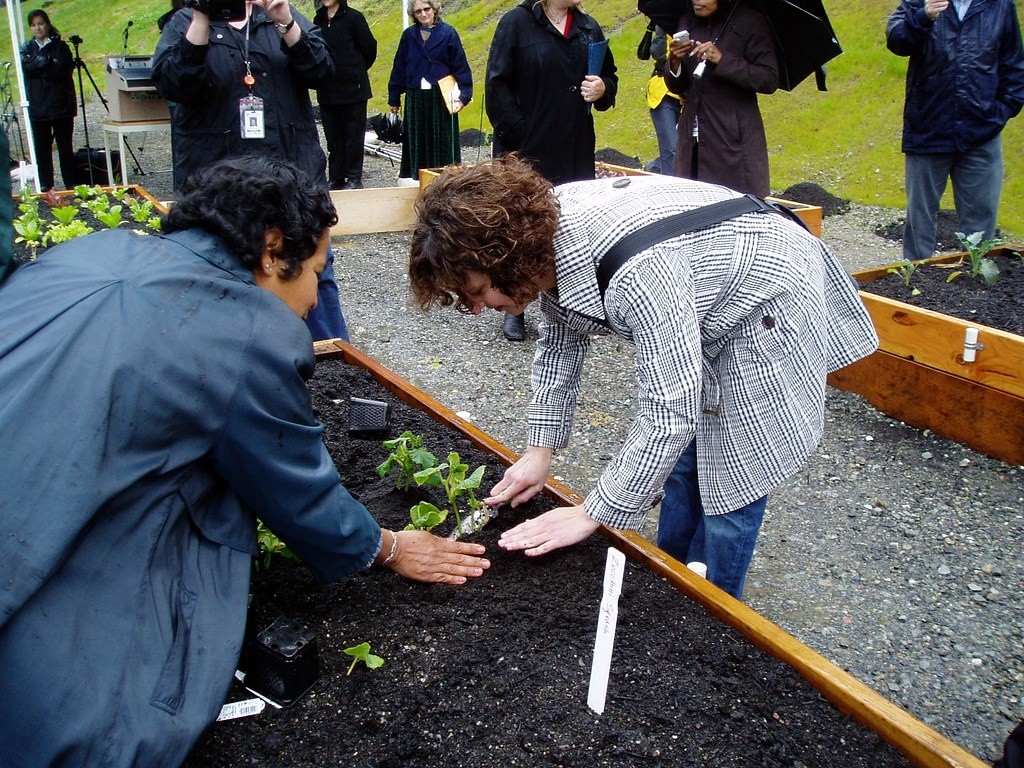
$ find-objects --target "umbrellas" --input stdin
[638,0,844,93]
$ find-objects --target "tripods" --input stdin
[66,34,145,188]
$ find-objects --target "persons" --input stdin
[251,119,257,127]
[885,0,1024,260]
[0,155,491,768]
[645,25,688,176]
[407,150,878,601]
[485,0,618,341]
[662,0,780,198]
[388,0,473,181]
[312,0,378,189]
[19,9,78,191]
[151,0,352,344]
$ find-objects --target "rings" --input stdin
[585,94,587,99]
[704,52,706,55]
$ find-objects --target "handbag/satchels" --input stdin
[75,147,122,186]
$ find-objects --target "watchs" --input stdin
[275,17,295,34]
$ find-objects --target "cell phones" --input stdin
[674,30,690,43]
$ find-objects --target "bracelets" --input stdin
[381,530,397,567]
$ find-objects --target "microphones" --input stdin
[121,21,134,35]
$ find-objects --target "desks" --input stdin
[102,121,172,186]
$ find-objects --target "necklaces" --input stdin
[545,7,566,24]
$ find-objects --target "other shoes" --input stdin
[40,186,51,193]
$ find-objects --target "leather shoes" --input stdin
[340,179,364,190]
[326,179,346,191]
[501,310,524,340]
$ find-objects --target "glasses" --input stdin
[414,5,434,15]
